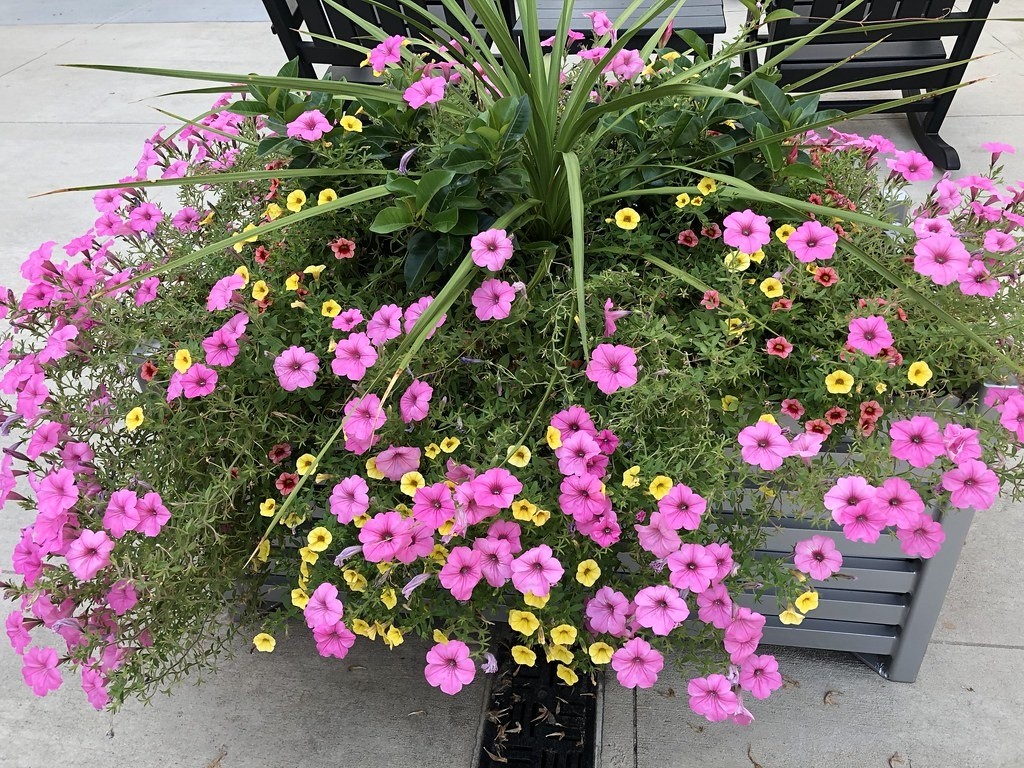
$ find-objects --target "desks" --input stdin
[510,0,727,64]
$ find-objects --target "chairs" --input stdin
[261,0,520,115]
[738,1,999,172]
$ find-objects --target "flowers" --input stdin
[0,0,1023,732]
